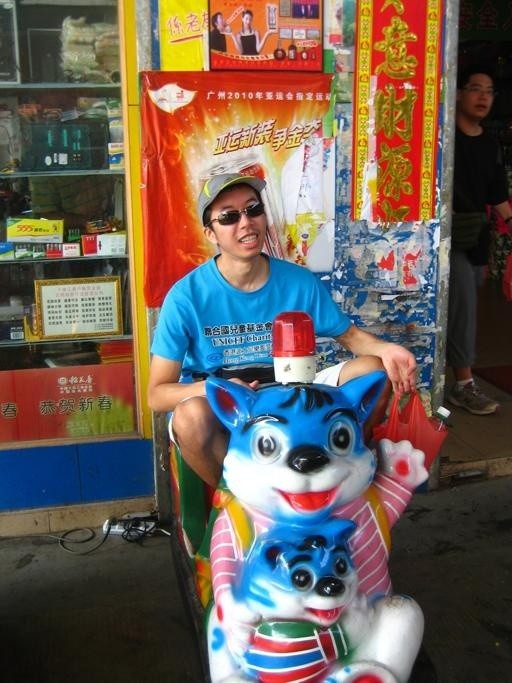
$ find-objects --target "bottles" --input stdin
[275,38,317,61]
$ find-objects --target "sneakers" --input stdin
[446,379,503,415]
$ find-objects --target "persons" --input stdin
[211,8,228,54]
[439,66,511,419]
[142,167,422,489]
[222,9,275,59]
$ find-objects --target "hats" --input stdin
[198,174,267,228]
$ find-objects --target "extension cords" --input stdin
[103,516,157,536]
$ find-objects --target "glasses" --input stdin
[206,203,264,225]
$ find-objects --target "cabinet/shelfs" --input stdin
[0,1,153,450]
